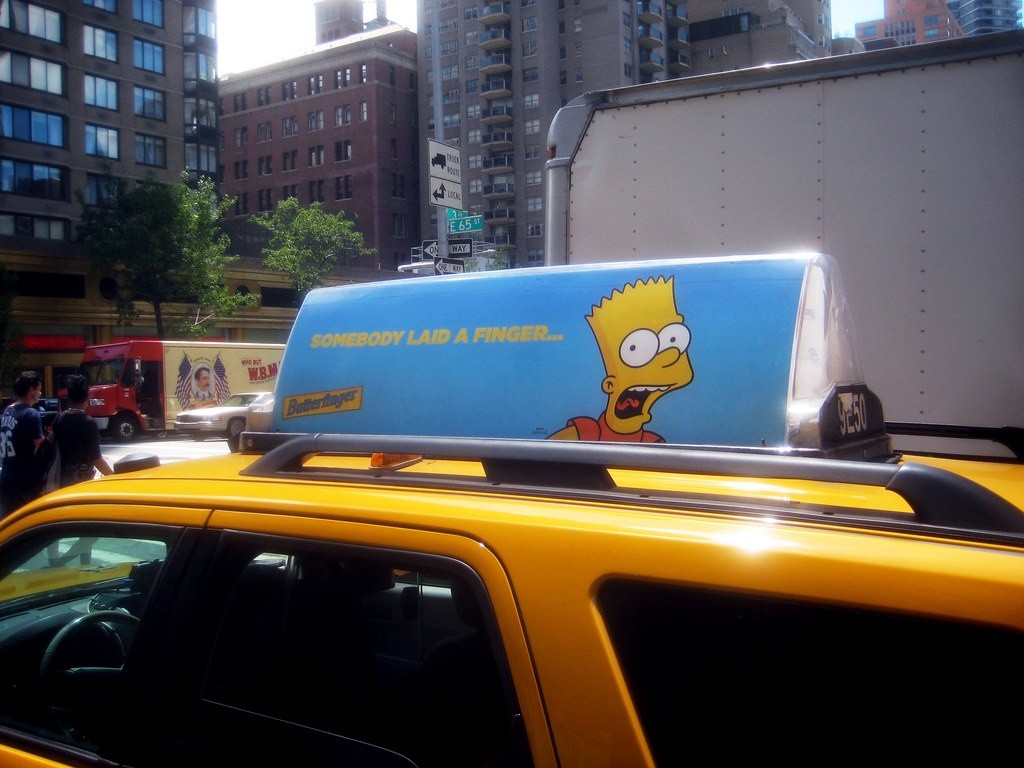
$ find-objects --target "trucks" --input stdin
[54,336,285,443]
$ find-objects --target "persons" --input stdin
[195,367,213,401]
[1,372,63,569]
[133,369,144,392]
[50,374,116,569]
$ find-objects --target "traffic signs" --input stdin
[422,137,483,276]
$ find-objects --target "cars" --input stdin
[0,242,1022,768]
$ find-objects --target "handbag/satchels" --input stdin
[20,439,62,504]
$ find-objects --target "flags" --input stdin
[214,351,231,404]
[175,349,193,411]
